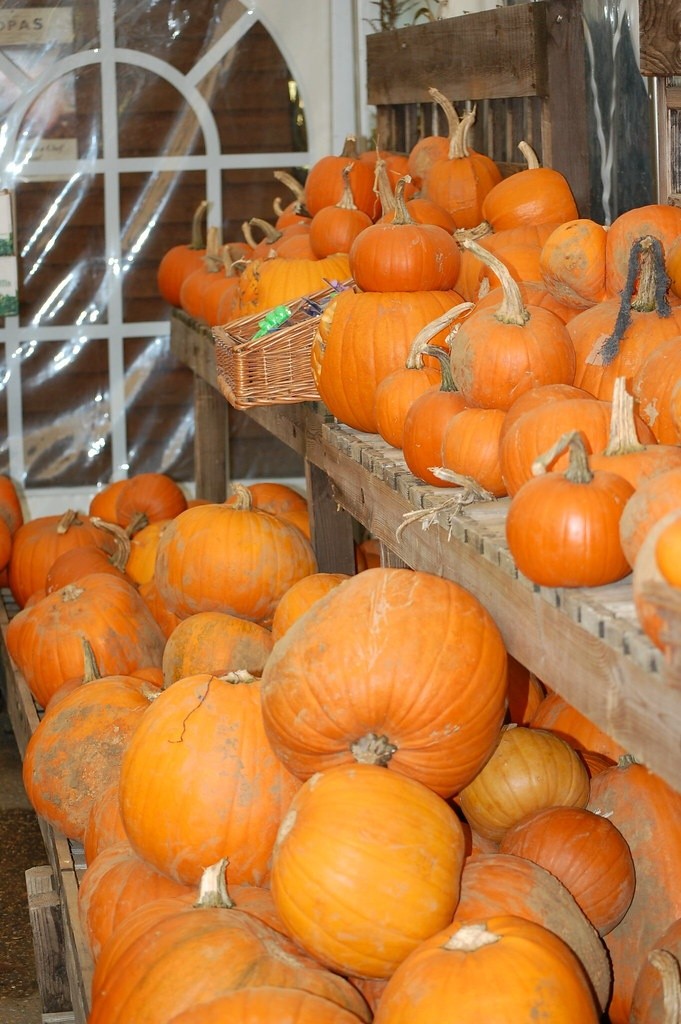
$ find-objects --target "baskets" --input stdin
[212,277,357,409]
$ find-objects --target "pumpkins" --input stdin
[0,84,681,1024]
